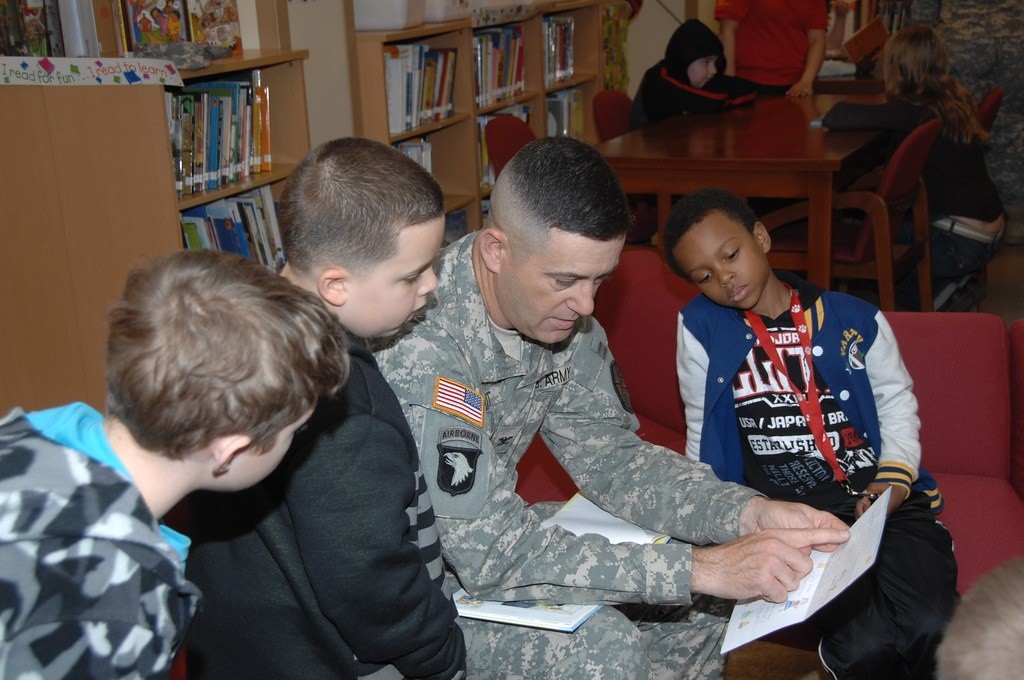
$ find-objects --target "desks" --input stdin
[595,94,894,294]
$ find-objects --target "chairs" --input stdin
[485,116,658,244]
[974,85,1005,154]
[751,116,940,313]
[593,88,634,141]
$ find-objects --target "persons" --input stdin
[0,249,346,679]
[630,16,771,130]
[911,0,1023,245]
[360,137,854,678]
[822,26,1008,311]
[657,190,961,678]
[714,2,828,97]
[153,137,466,679]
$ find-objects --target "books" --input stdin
[1,0,580,278]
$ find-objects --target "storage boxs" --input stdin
[472,0,535,28]
[424,0,472,23]
[354,0,425,32]
[1,53,188,420]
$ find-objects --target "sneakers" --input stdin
[926,289,972,312]
[957,277,990,311]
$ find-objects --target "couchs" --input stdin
[513,251,1023,616]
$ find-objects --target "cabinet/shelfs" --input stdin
[0,0,312,278]
[353,0,607,254]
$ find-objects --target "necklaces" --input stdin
[744,284,875,498]
